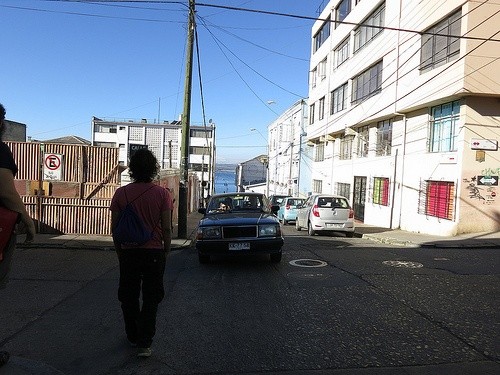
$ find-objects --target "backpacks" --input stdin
[114,185,161,248]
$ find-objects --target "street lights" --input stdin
[251,101,293,198]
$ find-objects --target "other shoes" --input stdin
[0,351,9,367]
[135,347,151,358]
[128,340,136,347]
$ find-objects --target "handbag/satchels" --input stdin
[0,206,21,261]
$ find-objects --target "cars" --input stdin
[268,194,307,225]
[296,195,355,238]
[195,192,285,265]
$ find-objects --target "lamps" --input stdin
[267,101,292,121]
[251,128,268,143]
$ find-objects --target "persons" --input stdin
[109,149,174,357]
[0,102,36,366]
